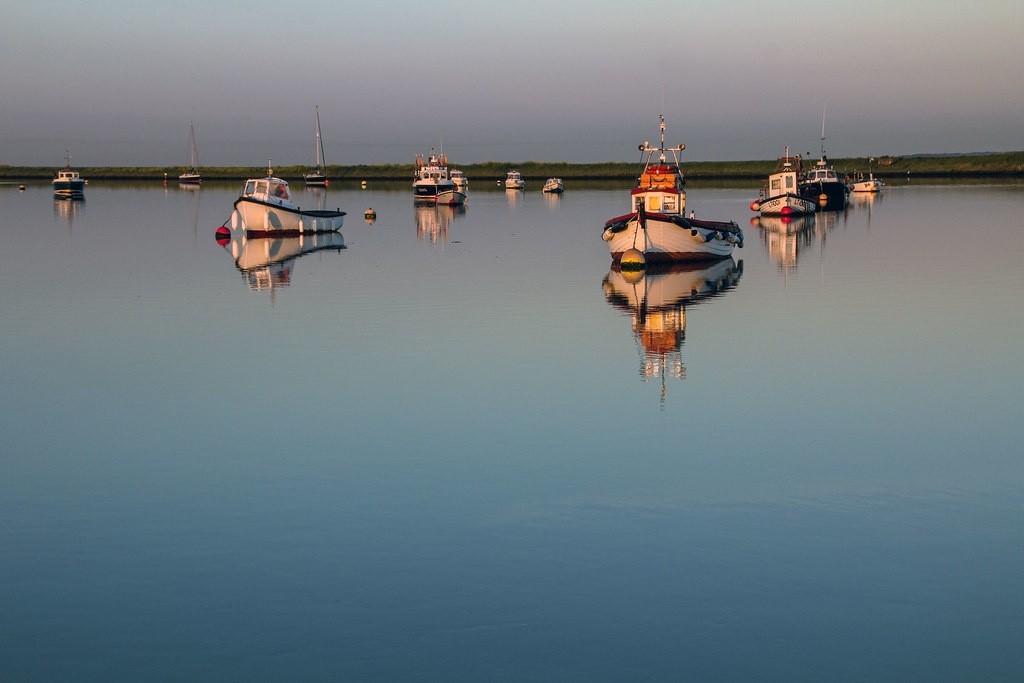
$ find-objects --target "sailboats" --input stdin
[300,104,331,183]
[178,120,204,183]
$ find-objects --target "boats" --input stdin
[841,162,883,192]
[411,137,449,202]
[448,168,467,187]
[213,234,347,291]
[794,106,856,212]
[51,168,89,196]
[435,187,467,204]
[214,158,347,234]
[506,170,526,188]
[600,256,745,411]
[749,145,817,215]
[602,110,745,266]
[542,176,567,193]
